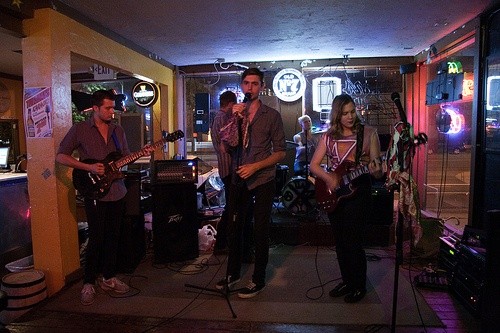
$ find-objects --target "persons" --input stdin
[211,91,256,264]
[309,94,387,301]
[56,89,156,307]
[215,67,286,298]
[293,114,317,174]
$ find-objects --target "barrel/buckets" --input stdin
[1,270,49,311]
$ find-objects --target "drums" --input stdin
[273,164,290,204]
[304,164,329,178]
[281,175,317,216]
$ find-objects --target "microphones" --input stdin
[243,92,251,104]
[391,91,408,126]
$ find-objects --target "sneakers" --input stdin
[344,286,367,304]
[215,277,235,289]
[329,282,350,298]
[238,280,264,299]
[81,283,96,305]
[101,277,129,293]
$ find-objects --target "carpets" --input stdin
[47,247,447,328]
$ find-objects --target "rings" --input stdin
[329,184,332,187]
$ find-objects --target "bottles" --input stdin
[26,109,35,137]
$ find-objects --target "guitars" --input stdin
[72,129,186,201]
[314,132,428,211]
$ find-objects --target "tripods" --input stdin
[184,120,249,318]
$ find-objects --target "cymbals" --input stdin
[272,140,299,151]
[312,130,326,135]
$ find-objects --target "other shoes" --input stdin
[213,248,229,256]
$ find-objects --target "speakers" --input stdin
[365,183,394,225]
[117,175,145,274]
[151,182,200,263]
